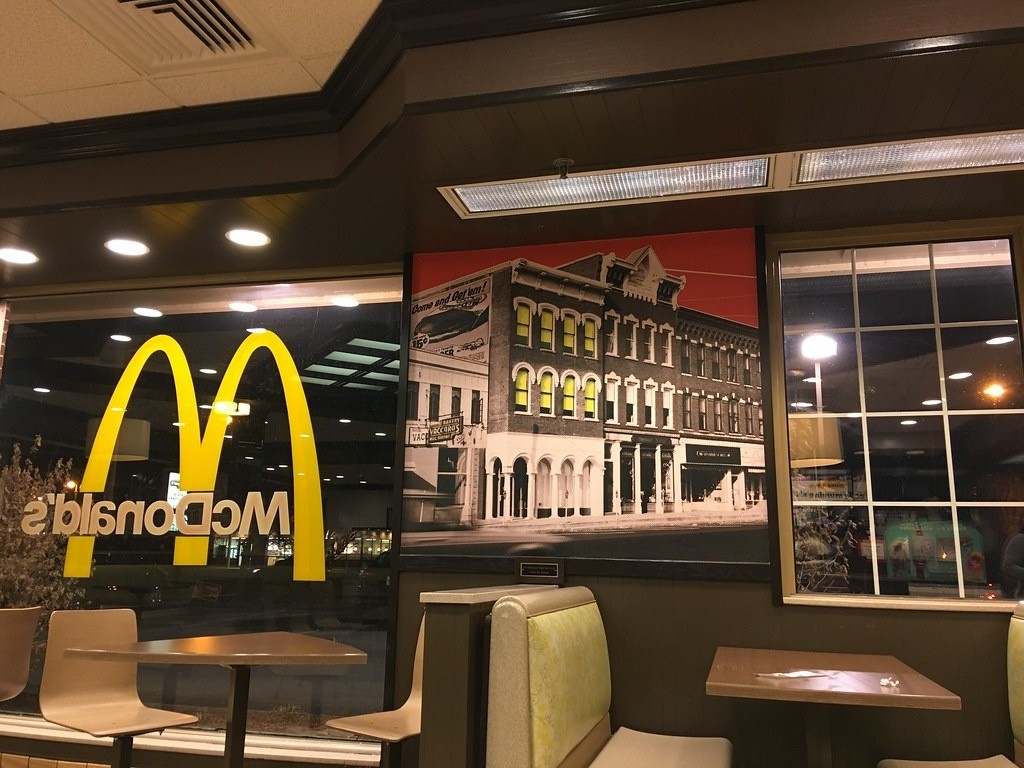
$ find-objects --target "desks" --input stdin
[705,646,962,768]
[64,631,369,768]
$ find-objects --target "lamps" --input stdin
[788,409,844,468]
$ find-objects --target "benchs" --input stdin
[490,585,734,768]
[881,601,1024,768]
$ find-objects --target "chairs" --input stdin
[0,606,42,703]
[322,611,424,768]
[38,610,199,768]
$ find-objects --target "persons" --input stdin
[1001,516,1024,599]
